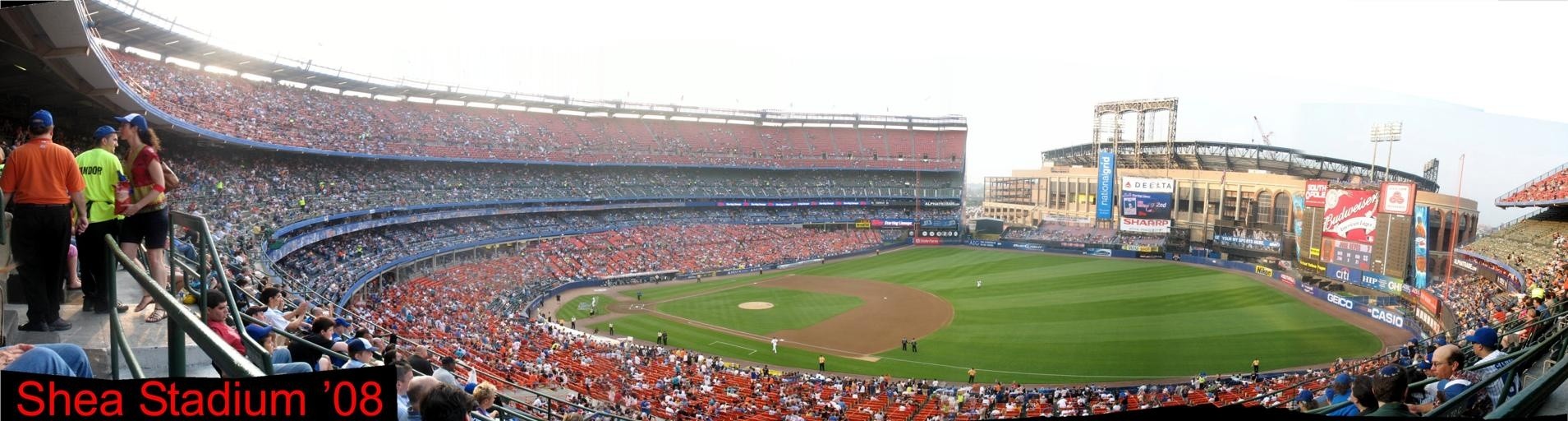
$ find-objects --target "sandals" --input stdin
[146,308,167,322]
[134,295,155,312]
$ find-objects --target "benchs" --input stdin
[363,225,1327,421]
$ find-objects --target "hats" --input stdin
[245,306,268,315]
[348,337,379,355]
[114,113,148,134]
[464,383,477,395]
[245,323,272,339]
[94,125,121,140]
[32,110,53,127]
[442,356,459,364]
[336,318,351,327]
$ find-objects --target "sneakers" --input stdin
[18,321,49,332]
[49,317,72,332]
[82,301,128,313]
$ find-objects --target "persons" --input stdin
[1,48,1568,420]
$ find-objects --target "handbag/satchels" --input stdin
[162,161,179,193]
